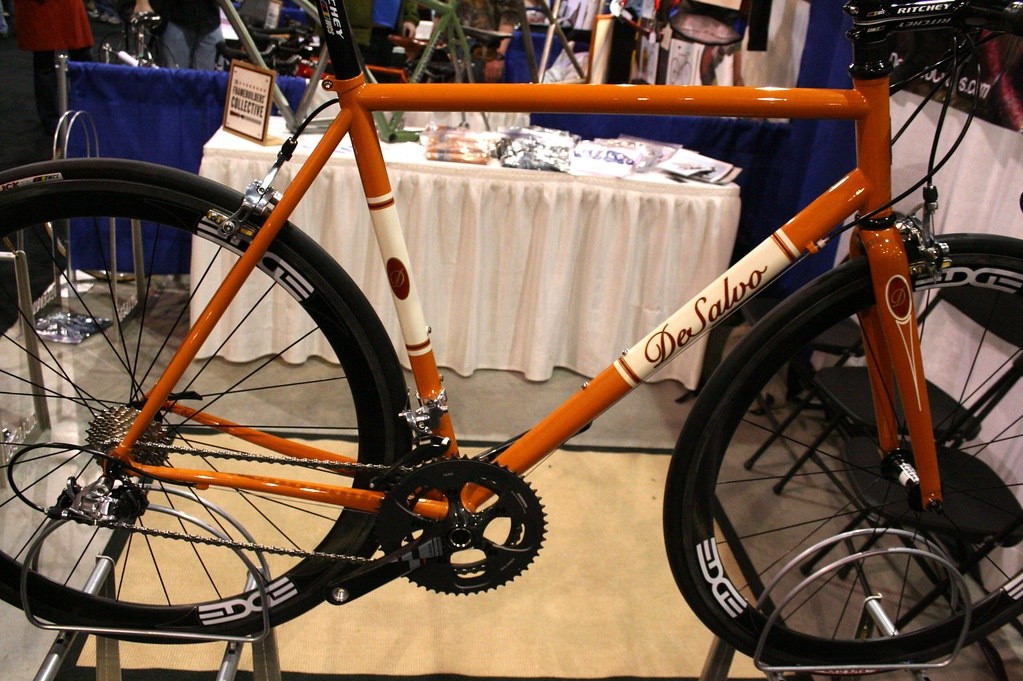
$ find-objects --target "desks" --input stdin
[188,116,743,390]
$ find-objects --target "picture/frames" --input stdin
[223,58,276,142]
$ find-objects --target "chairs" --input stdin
[746,267,1023,681]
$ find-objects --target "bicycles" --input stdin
[0,0,1023,678]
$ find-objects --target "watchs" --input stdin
[495,52,505,60]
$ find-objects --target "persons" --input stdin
[430,0,514,83]
[344,0,420,83]
[134,0,226,70]
[15,0,94,138]
